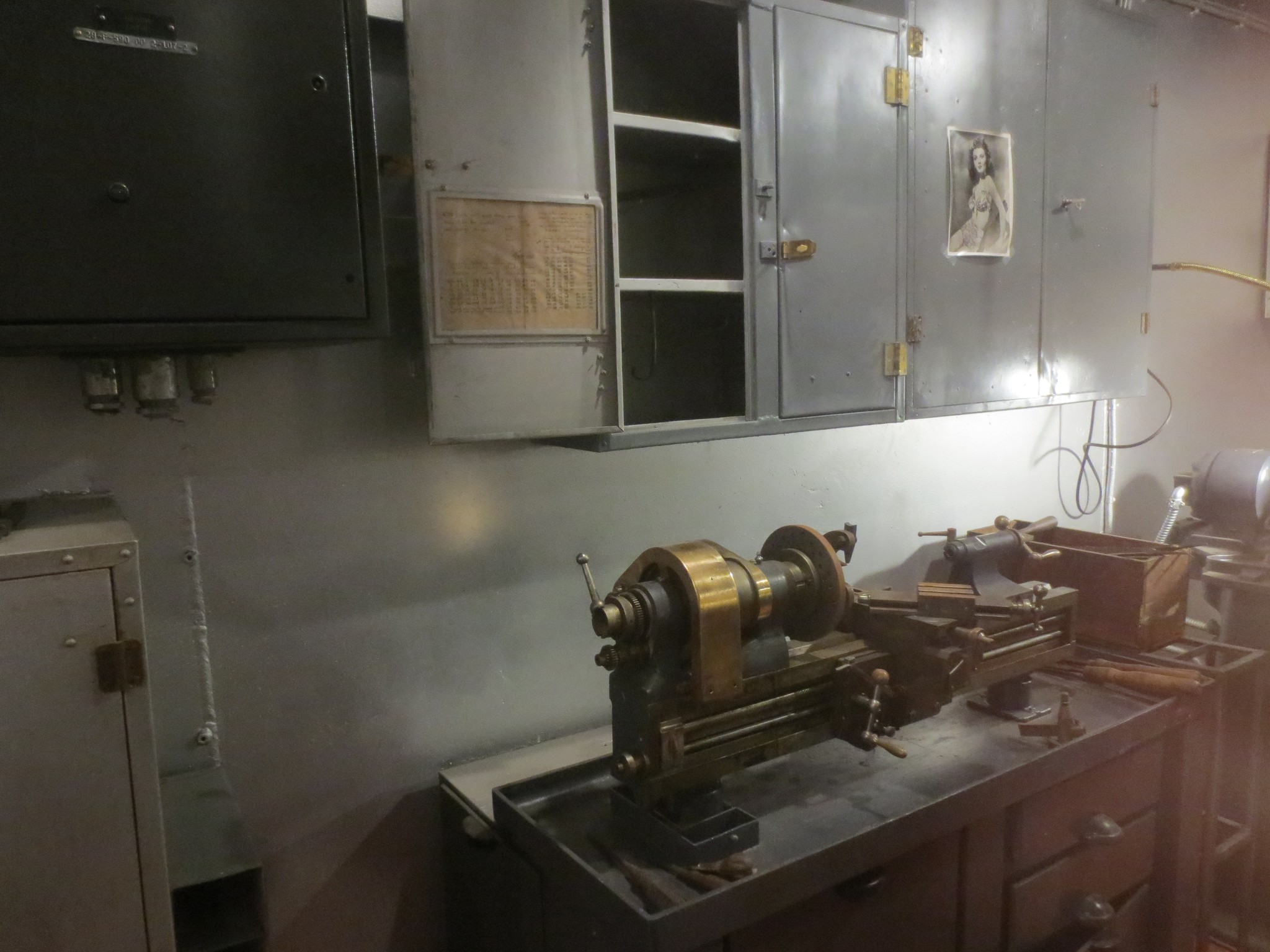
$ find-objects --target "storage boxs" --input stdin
[967,518,1193,653]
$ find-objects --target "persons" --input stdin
[949,137,1009,254]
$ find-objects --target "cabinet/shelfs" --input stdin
[909,665,1186,952]
[436,673,964,952]
[900,0,1163,421]
[402,0,903,454]
[0,490,183,951]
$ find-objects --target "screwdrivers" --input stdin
[585,831,755,915]
